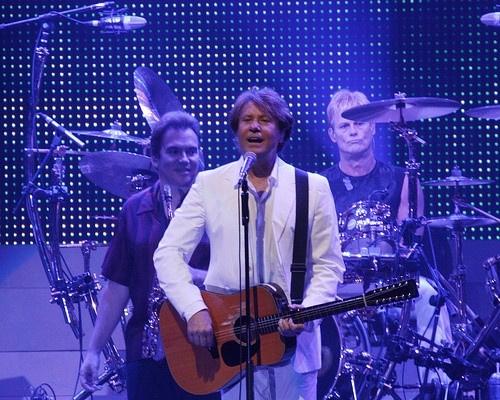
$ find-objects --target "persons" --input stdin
[80,110,223,400]
[318,89,455,385]
[153,87,346,400]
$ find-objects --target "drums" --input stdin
[316,300,371,395]
[338,200,401,262]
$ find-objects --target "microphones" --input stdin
[40,114,85,148]
[88,15,147,30]
[163,184,173,220]
[238,151,257,185]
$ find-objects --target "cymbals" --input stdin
[422,215,498,231]
[68,120,148,147]
[481,10,499,28]
[465,102,500,121]
[340,94,461,122]
[26,143,90,156]
[79,151,158,201]
[422,166,499,189]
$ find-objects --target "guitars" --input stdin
[154,280,422,396]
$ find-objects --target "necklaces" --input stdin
[335,161,380,191]
